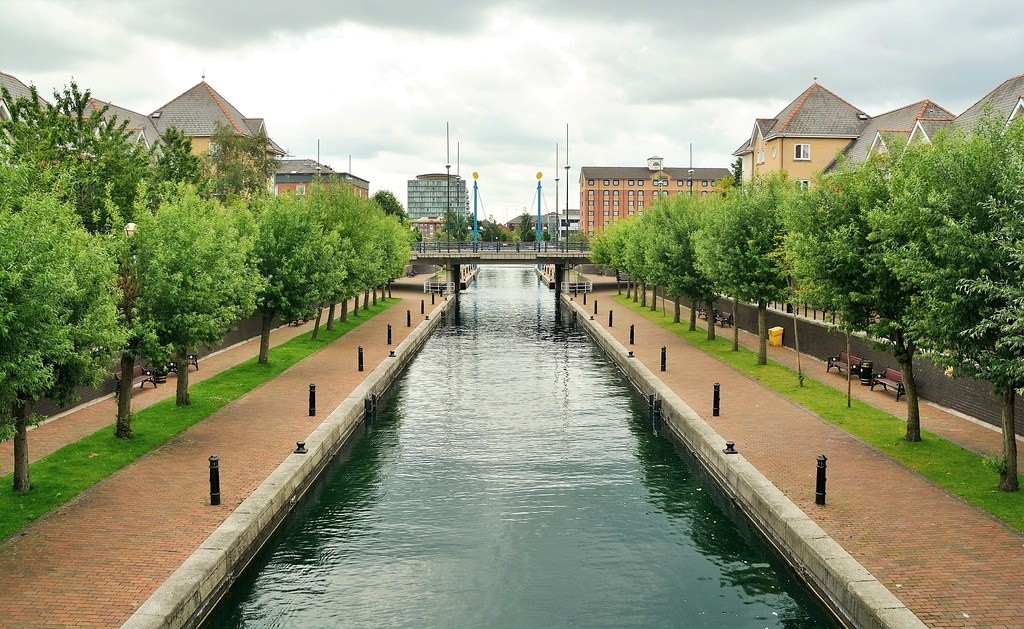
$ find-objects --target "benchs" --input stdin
[870,365,917,402]
[826,350,864,381]
[289,316,308,326]
[164,352,199,375]
[698,307,719,321]
[713,311,733,328]
[112,362,157,399]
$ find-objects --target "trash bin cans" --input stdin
[768,326,784,347]
